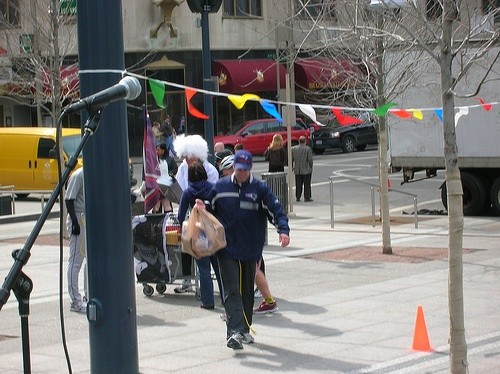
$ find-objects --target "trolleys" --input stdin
[133,215,201,301]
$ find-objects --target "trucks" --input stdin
[384,41,500,214]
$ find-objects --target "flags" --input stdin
[142,110,160,213]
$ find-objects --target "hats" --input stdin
[180,116,186,120]
[234,150,252,172]
[165,115,170,118]
[214,151,229,159]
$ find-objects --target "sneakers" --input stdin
[174,280,193,293]
[220,312,228,321]
[253,300,279,314]
[243,332,255,344]
[70,300,87,313]
[227,333,243,349]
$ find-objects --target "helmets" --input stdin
[219,155,234,170]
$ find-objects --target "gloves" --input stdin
[72,225,81,236]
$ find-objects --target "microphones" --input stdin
[65,76,142,113]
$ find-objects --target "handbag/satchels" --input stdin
[180,205,227,259]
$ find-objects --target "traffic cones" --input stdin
[388,173,390,192]
[411,305,431,351]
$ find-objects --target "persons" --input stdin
[140,113,279,314]
[263,133,288,172]
[292,135,314,202]
[197,150,289,350]
[65,166,87,313]
[253,124,261,134]
[130,178,141,204]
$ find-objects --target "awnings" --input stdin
[294,58,362,89]
[212,57,288,93]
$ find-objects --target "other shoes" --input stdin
[304,199,312,202]
[201,304,214,309]
[296,199,301,201]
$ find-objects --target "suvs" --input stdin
[213,118,311,165]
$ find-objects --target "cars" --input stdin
[312,111,378,155]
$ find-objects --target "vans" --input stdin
[1,128,137,206]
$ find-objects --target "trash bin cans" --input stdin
[261,172,288,215]
